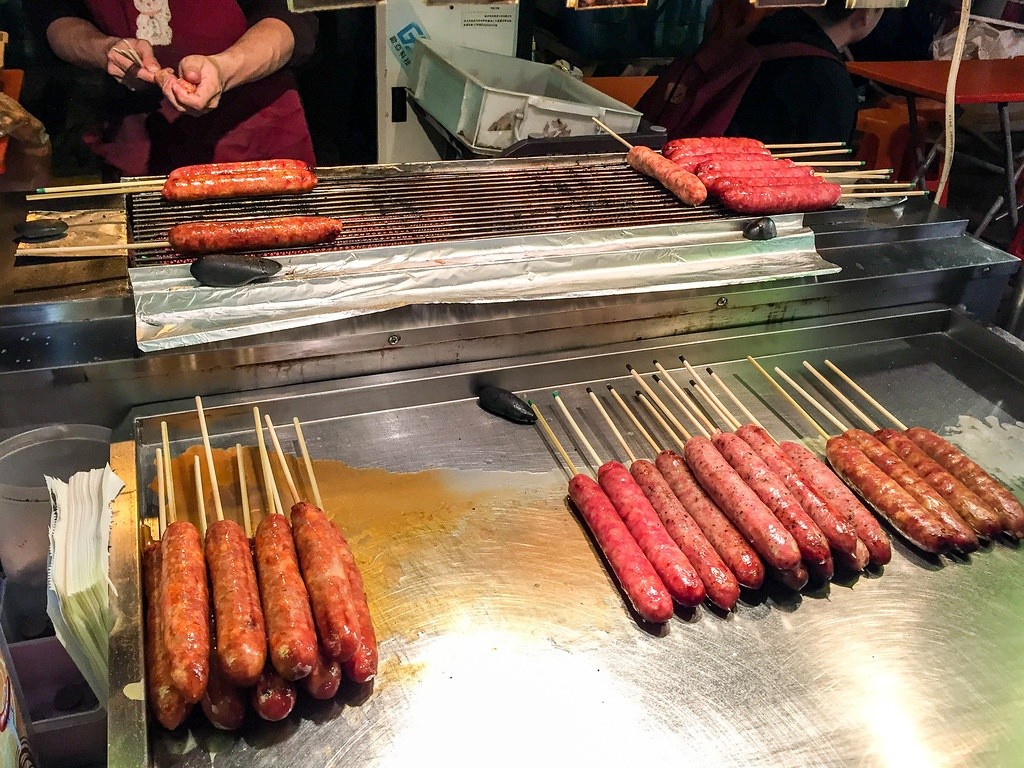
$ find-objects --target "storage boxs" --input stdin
[404,36,645,152]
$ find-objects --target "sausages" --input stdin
[158,160,343,256]
[565,425,1023,623]
[624,138,841,217]
[144,503,380,731]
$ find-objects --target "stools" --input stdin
[850,108,926,185]
[876,92,951,208]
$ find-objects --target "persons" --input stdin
[33,1,321,180]
[681,0,884,169]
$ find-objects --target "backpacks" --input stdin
[632,14,846,143]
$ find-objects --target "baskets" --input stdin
[408,37,644,150]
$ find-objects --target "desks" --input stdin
[845,54,1024,243]
